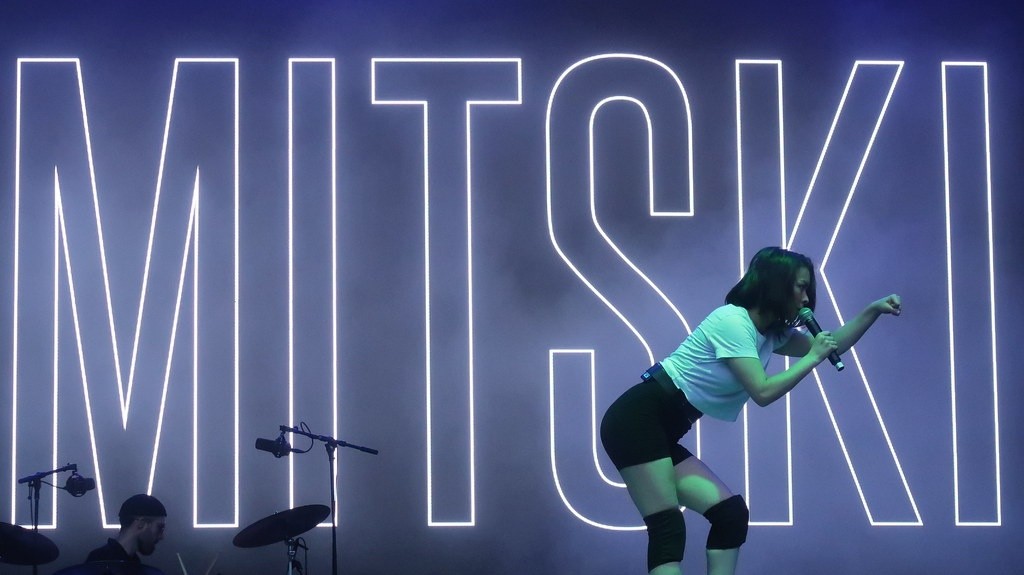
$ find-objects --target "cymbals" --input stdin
[232,504,331,549]
[0,520,61,567]
[52,558,164,575]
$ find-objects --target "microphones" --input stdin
[799,307,844,372]
[64,478,96,492]
[287,538,300,575]
[256,438,305,458]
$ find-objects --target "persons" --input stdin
[600,247,902,575]
[85,494,168,563]
